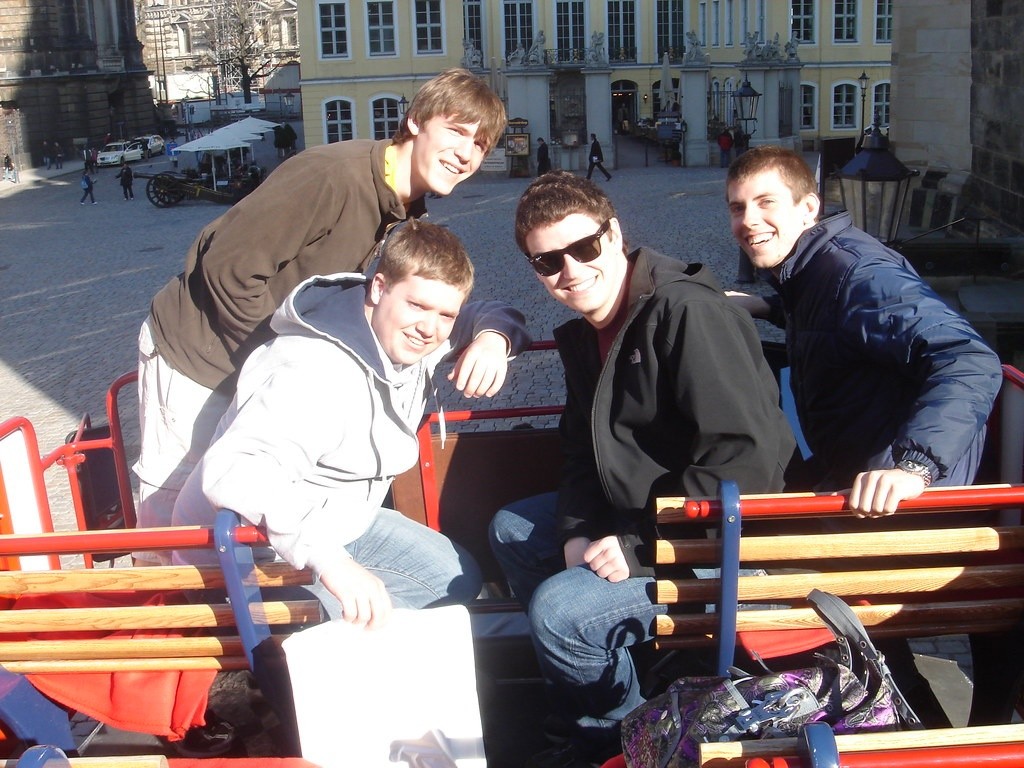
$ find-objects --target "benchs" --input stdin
[1,342,1024,768]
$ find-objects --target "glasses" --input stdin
[524,219,610,276]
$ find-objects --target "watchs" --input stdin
[895,460,931,487]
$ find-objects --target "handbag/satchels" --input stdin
[621,585,927,768]
[80,182,88,188]
[592,156,602,162]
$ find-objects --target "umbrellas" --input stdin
[170,131,253,191]
[659,51,675,112]
[208,116,283,177]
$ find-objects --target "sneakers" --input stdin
[167,709,240,761]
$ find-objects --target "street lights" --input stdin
[855,70,871,154]
[730,71,762,285]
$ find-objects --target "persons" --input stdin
[463,37,482,67]
[786,31,803,61]
[80,172,98,205]
[170,224,529,629]
[491,169,798,768]
[586,133,612,182]
[618,102,630,137]
[589,30,604,62]
[130,67,507,568]
[82,145,99,175]
[2,153,14,179]
[41,141,64,171]
[113,163,134,201]
[274,121,297,158]
[717,125,744,168]
[140,138,149,161]
[512,31,545,65]
[536,137,549,177]
[726,145,1005,521]
[685,32,704,60]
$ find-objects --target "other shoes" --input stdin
[606,176,612,181]
[93,202,98,205]
[80,202,84,204]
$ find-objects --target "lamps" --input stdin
[284,92,302,115]
[398,94,410,114]
[644,95,647,103]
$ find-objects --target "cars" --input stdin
[132,134,166,158]
[96,140,142,167]
